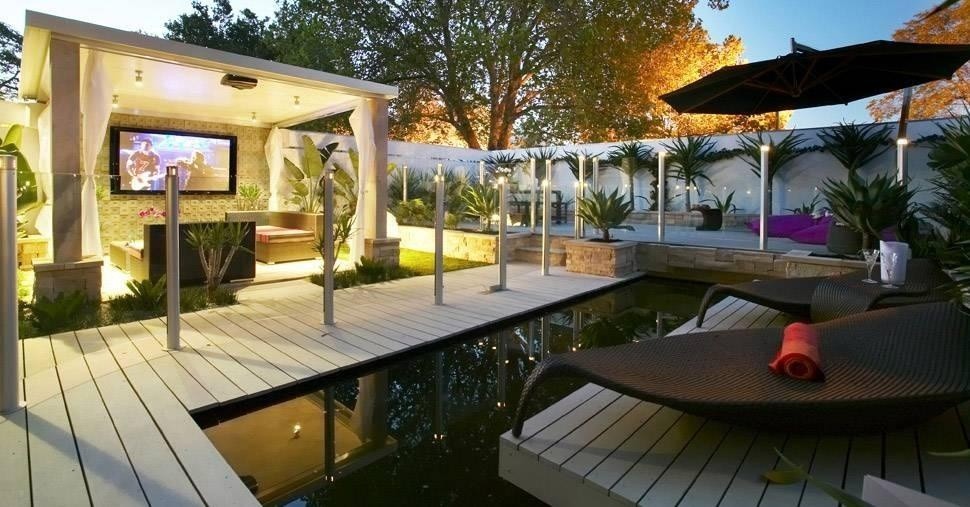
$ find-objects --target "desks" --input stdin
[695,253,964,330]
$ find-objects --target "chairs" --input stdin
[512,284,970,438]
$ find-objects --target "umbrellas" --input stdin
[658,39,970,116]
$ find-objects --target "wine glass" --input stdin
[880,251,900,288]
[860,248,881,284]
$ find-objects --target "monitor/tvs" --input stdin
[110,124,237,195]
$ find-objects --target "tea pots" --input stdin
[879,239,913,285]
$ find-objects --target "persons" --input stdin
[177,151,214,191]
[126,138,160,190]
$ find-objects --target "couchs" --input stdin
[226,209,324,266]
[128,222,256,286]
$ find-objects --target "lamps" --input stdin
[112,94,119,108]
[252,112,257,122]
[294,96,300,109]
[135,70,144,87]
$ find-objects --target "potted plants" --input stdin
[562,186,640,278]
